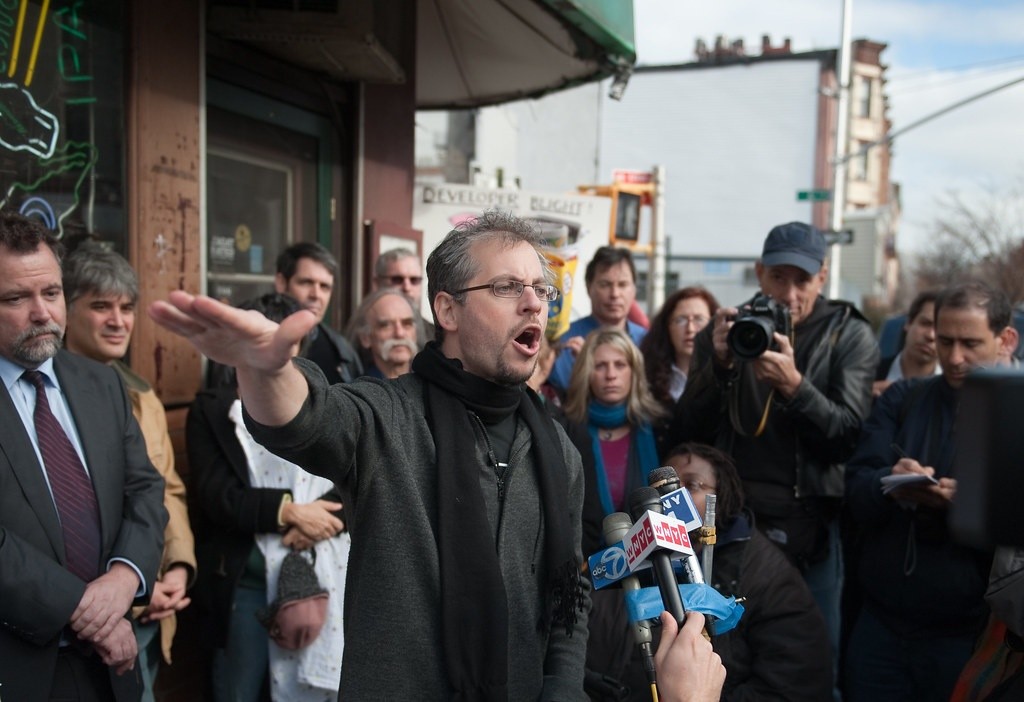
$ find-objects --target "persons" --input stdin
[184,220,1024,702]
[0,212,171,702]
[62,239,199,702]
[652,609,726,702]
[147,210,593,702]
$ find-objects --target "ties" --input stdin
[21,371,102,582]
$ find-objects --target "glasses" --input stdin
[666,316,710,328]
[380,275,424,286]
[450,281,561,302]
[681,479,718,492]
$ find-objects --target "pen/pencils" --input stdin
[889,443,909,458]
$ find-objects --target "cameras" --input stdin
[727,295,789,361]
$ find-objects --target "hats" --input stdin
[763,222,826,276]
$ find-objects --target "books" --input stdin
[879,473,942,511]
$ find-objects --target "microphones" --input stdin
[622,487,694,633]
[647,466,716,637]
[588,513,657,684]
